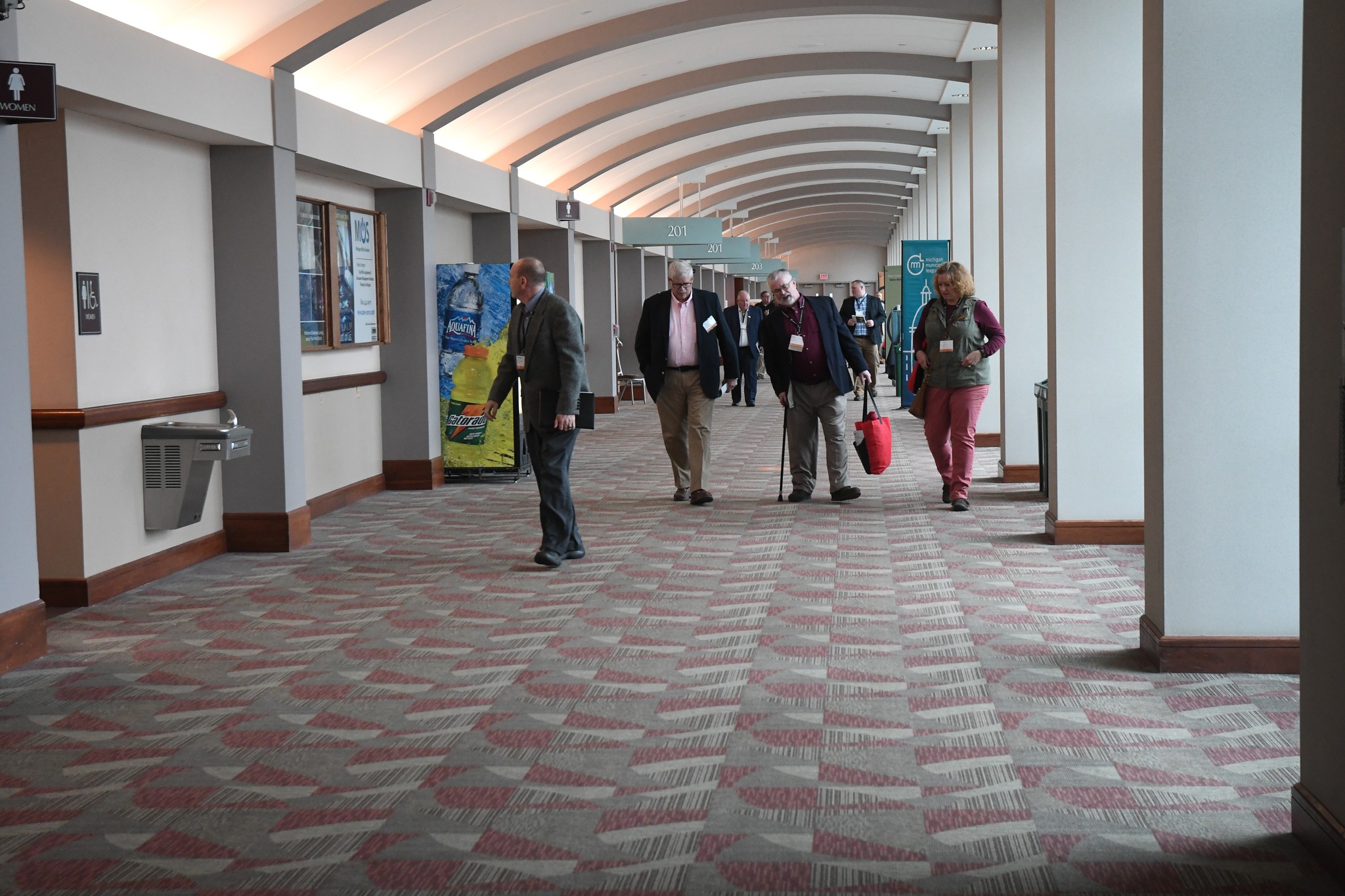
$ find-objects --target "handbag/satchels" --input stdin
[908,383,926,419]
[853,380,892,474]
[907,339,928,393]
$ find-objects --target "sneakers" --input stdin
[942,483,952,503]
[854,394,864,400]
[951,498,970,512]
[870,388,877,396]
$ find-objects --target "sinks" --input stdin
[152,421,245,432]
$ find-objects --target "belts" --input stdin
[853,335,870,339]
[667,365,698,373]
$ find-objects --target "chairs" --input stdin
[616,347,646,405]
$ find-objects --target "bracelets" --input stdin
[980,349,986,358]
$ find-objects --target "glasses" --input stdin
[670,277,693,288]
[763,295,771,298]
[773,279,793,295]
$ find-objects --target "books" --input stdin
[538,391,594,430]
[852,315,867,323]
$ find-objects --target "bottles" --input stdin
[445,344,494,463]
[439,264,484,378]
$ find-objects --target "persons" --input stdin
[484,257,590,566]
[761,269,872,502]
[839,280,886,401]
[634,259,739,505]
[875,292,885,364]
[754,291,779,380]
[912,261,1005,511]
[723,291,764,406]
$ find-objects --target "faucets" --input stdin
[226,409,238,427]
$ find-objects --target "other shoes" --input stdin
[560,545,586,559]
[732,401,739,406]
[879,361,883,364]
[746,402,755,407]
[534,547,561,566]
[757,376,765,380]
[848,366,852,368]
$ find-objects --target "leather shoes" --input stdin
[691,489,713,505]
[674,486,691,500]
[832,485,861,501]
[789,490,811,502]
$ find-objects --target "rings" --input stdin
[568,426,572,428]
[968,365,971,367]
